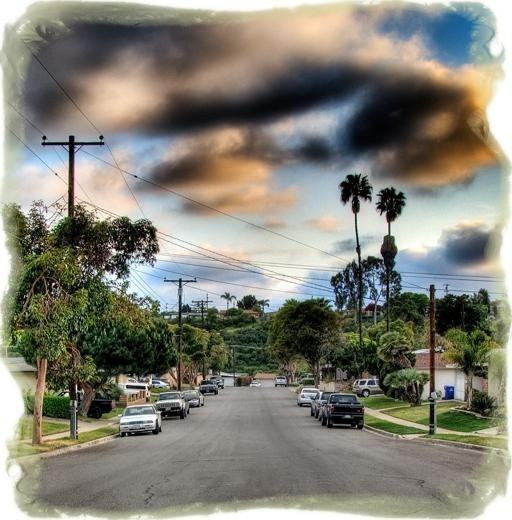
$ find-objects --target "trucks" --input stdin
[118,383,151,402]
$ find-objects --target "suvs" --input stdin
[298,373,321,385]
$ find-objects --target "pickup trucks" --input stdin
[56,391,115,419]
[322,393,364,429]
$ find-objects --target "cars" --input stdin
[274,376,287,388]
[295,387,324,407]
[198,380,219,395]
[309,392,342,421]
[180,390,205,407]
[139,374,160,382]
[140,378,171,391]
[318,401,327,421]
[127,376,139,383]
[207,376,225,390]
[118,404,162,437]
[249,380,262,388]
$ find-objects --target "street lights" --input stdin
[231,346,235,387]
[201,332,223,380]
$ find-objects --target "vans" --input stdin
[154,391,190,419]
[352,378,384,397]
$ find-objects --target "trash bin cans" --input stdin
[444,384,454,400]
